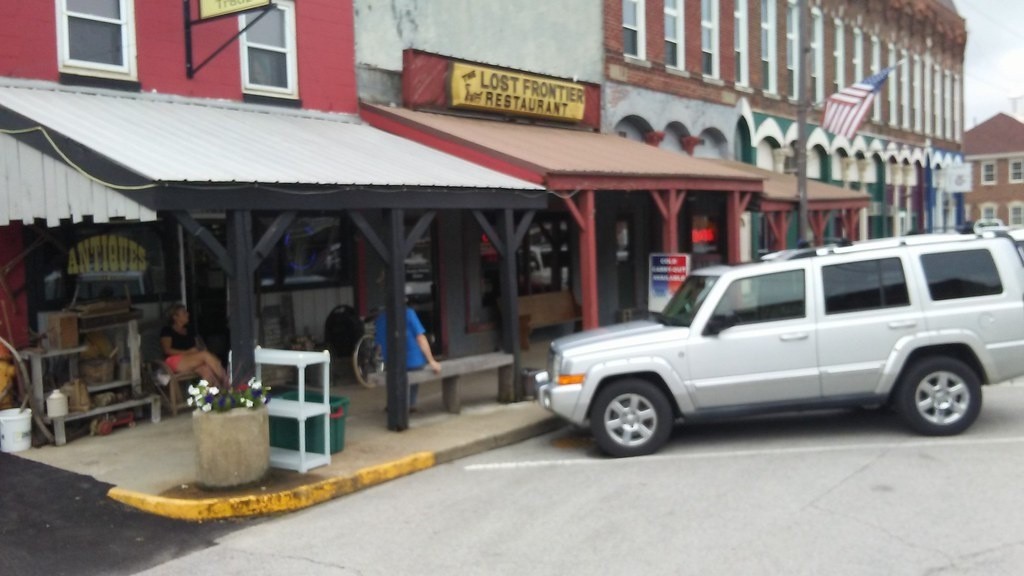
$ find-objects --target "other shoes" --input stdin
[234,384,250,393]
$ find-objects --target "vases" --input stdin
[192,408,270,487]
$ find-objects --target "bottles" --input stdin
[46,389,68,418]
[151,395,162,422]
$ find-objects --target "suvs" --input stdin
[524,229,1024,460]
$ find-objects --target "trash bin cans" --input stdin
[522,367,539,401]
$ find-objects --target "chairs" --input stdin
[140,334,203,415]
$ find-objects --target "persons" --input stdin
[373,293,442,413]
[159,302,227,387]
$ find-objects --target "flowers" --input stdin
[185,377,272,413]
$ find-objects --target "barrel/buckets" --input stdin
[0,408,32,454]
[79,354,115,385]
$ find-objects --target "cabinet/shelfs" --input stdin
[31,308,142,440]
[253,346,330,474]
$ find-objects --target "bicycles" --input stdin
[352,312,391,389]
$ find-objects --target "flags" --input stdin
[820,69,886,137]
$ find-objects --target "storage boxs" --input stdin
[269,392,349,454]
[268,384,293,446]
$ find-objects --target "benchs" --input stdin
[367,350,514,412]
[49,393,161,446]
[495,290,582,350]
